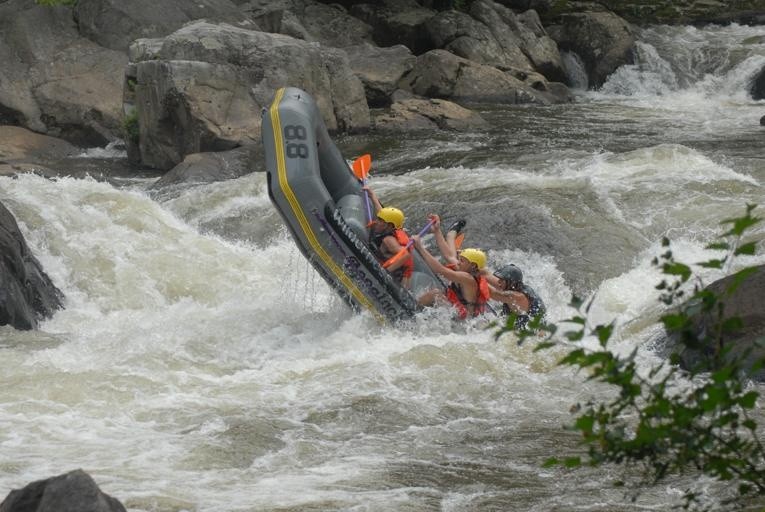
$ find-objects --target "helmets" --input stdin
[460,248,486,270]
[492,264,522,282]
[376,206,404,229]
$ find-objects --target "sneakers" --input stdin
[444,220,466,240]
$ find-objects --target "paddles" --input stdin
[383,215,438,268]
[353,154,377,227]
[456,233,464,249]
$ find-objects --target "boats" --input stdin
[259,85,501,335]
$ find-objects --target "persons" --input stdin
[360,184,415,293]
[483,262,546,337]
[410,213,488,325]
